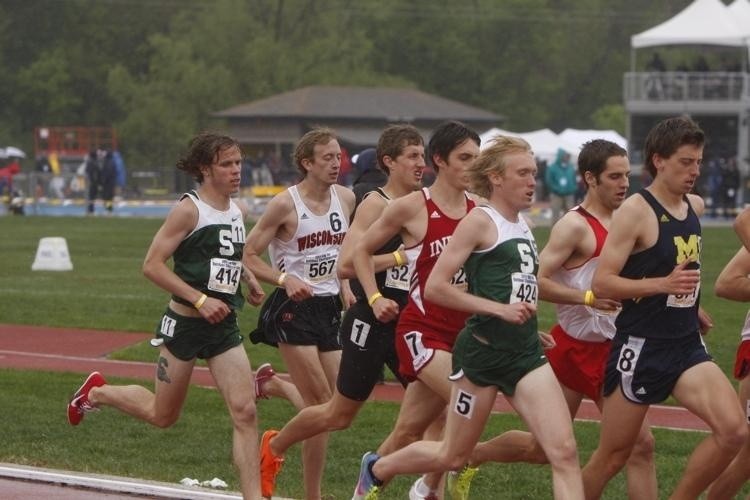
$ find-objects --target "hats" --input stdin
[356,149,377,170]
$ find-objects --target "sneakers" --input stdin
[447,467,479,500]
[351,452,384,500]
[259,431,285,500]
[409,479,445,500]
[253,363,276,404]
[67,372,107,424]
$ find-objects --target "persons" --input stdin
[0,160,29,215]
[644,49,743,99]
[579,114,749,500]
[241,123,356,500]
[543,147,577,228]
[34,143,128,216]
[351,133,585,500]
[705,155,739,219]
[350,119,482,499]
[446,139,657,500]
[732,202,750,251]
[705,246,750,500]
[349,147,409,393]
[259,125,448,500]
[66,132,267,500]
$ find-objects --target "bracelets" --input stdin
[278,272,287,287]
[194,292,208,311]
[584,289,594,307]
[367,291,383,307]
[393,250,403,268]
